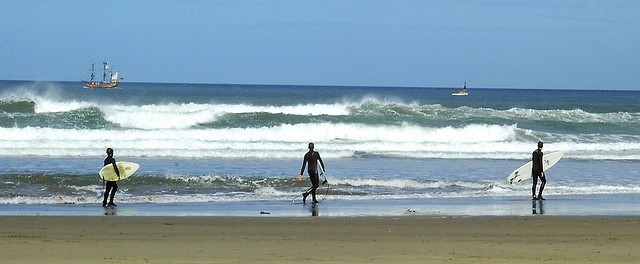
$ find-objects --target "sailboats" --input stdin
[86,63,118,88]
[452,81,468,95]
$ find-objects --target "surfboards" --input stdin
[316,160,329,185]
[506,151,564,185]
[98,161,139,182]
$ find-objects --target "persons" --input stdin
[531,142,545,199]
[299,142,325,204]
[102,148,120,207]
